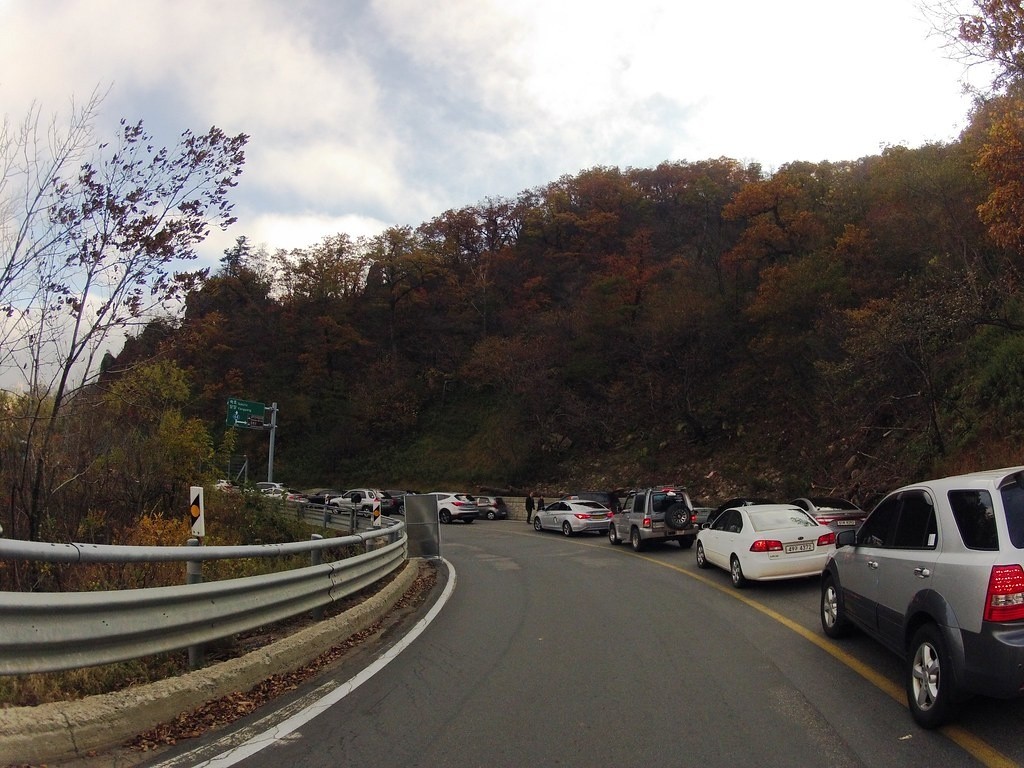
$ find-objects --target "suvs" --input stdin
[216,479,241,495]
[608,484,701,553]
[820,464,1024,729]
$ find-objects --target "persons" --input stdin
[537,494,544,512]
[606,483,622,514]
[525,491,535,525]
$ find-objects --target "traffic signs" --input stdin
[226,398,266,429]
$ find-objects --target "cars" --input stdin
[694,493,871,587]
[257,480,305,504]
[533,491,623,538]
[306,487,510,524]
